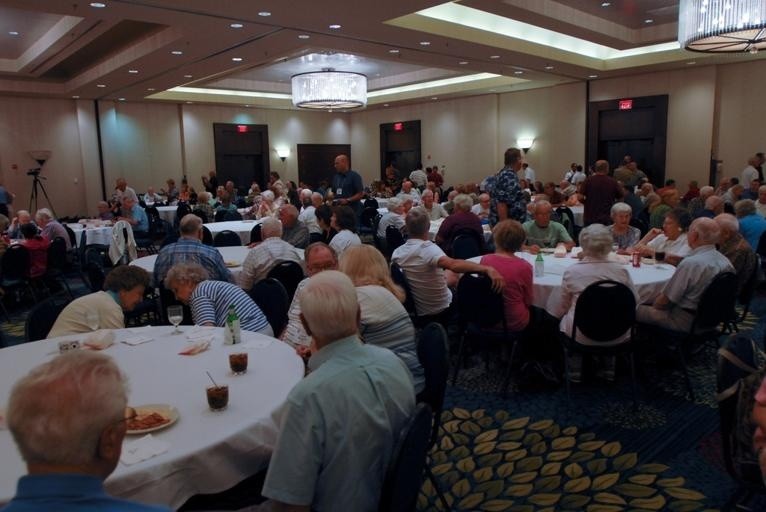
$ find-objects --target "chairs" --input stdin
[391,263,449,330]
[27,236,75,304]
[66,231,92,293]
[192,209,208,224]
[414,322,458,451]
[266,260,304,303]
[26,295,71,341]
[386,224,406,255]
[363,198,379,209]
[87,260,162,327]
[449,233,487,260]
[250,222,263,243]
[739,253,762,324]
[132,208,161,256]
[214,229,242,246]
[358,206,378,236]
[640,272,739,404]
[0,244,38,324]
[556,279,637,412]
[376,403,453,512]
[450,270,536,398]
[250,278,290,338]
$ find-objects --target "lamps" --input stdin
[291,70,369,112]
[676,1,766,54]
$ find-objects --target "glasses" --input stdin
[108,408,138,429]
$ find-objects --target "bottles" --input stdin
[633,252,642,268]
[535,251,545,278]
[224,304,241,344]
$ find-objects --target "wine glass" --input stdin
[654,248,666,270]
[167,304,184,335]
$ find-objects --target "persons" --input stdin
[277,204,311,250]
[0,351,174,512]
[619,185,642,222]
[298,198,325,243]
[12,223,49,309]
[143,186,165,208]
[655,179,675,195]
[435,194,484,257]
[154,213,234,290]
[215,185,226,205]
[648,189,680,230]
[192,192,214,223]
[740,179,760,200]
[576,159,625,227]
[314,204,338,246]
[0,183,16,222]
[741,156,759,189]
[544,223,643,388]
[413,189,450,220]
[604,202,641,250]
[93,200,115,222]
[750,375,766,486]
[45,265,150,340]
[396,193,413,219]
[216,193,238,221]
[163,261,275,338]
[426,181,441,203]
[571,165,587,187]
[712,213,756,321]
[425,167,441,188]
[236,216,308,293]
[722,185,745,214]
[563,163,577,182]
[477,219,547,376]
[716,177,732,196]
[311,192,324,208]
[687,186,714,215]
[432,165,444,185]
[332,154,364,217]
[520,200,576,254]
[755,184,766,216]
[613,152,633,185]
[160,179,180,206]
[628,162,647,186]
[635,217,736,388]
[317,180,328,195]
[688,196,724,233]
[755,152,766,184]
[329,206,362,264]
[376,196,407,256]
[488,147,527,230]
[109,177,139,215]
[34,208,74,297]
[238,269,416,512]
[519,180,541,196]
[277,241,340,370]
[339,245,429,417]
[116,194,150,239]
[7,209,39,240]
[634,207,692,267]
[470,193,491,224]
[391,206,506,369]
[185,187,198,205]
[225,181,238,202]
[679,180,700,205]
[298,188,312,214]
[522,163,535,184]
[544,181,564,204]
[201,169,219,199]
[441,190,460,215]
[249,172,300,217]
[560,179,577,197]
[396,180,420,201]
[734,198,766,252]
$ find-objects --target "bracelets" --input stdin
[348,197,353,203]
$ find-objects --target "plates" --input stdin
[126,403,179,433]
[222,261,241,267]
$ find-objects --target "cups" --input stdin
[228,342,249,376]
[206,376,230,413]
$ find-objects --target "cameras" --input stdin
[26,168,42,177]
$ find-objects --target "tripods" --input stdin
[27,176,59,222]
